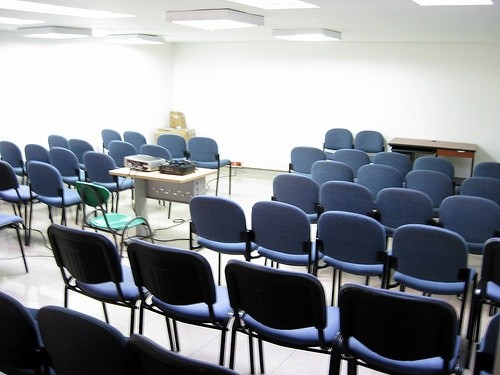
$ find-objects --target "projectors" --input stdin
[122,154,167,172]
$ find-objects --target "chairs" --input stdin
[0,128,500,375]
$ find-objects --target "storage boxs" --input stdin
[155,129,194,149]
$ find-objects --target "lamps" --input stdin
[18,28,92,39]
[273,28,341,42]
[107,34,163,46]
[166,8,265,30]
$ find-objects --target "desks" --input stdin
[389,137,478,185]
[109,167,218,254]
